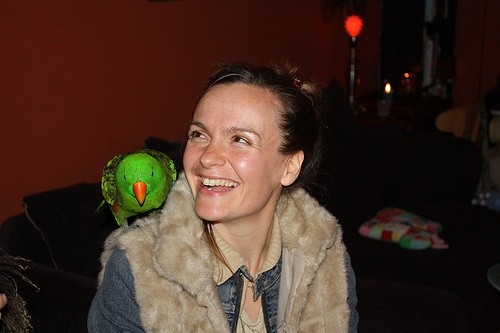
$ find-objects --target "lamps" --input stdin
[341,14,366,120]
[375,82,393,118]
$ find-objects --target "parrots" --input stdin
[95,150,178,228]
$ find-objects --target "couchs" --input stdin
[2,134,500,332]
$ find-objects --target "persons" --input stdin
[86,61,360,333]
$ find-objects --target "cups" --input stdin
[376,99,392,117]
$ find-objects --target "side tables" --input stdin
[347,91,453,137]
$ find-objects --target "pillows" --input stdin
[20,182,120,283]
[144,136,187,174]
